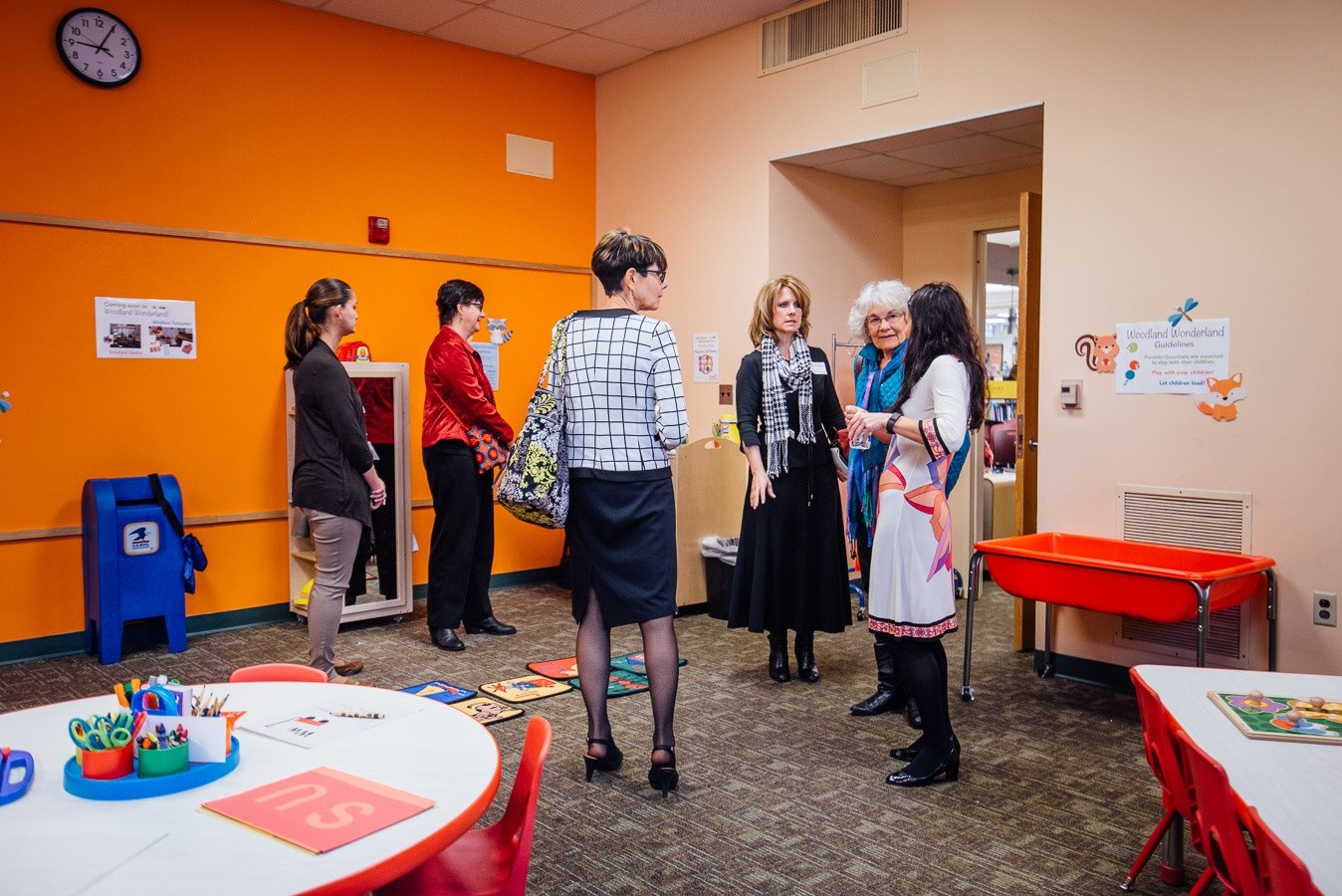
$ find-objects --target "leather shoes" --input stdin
[850,685,906,715]
[465,616,517,635]
[906,696,924,729]
[430,628,466,651]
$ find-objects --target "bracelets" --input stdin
[886,412,902,434]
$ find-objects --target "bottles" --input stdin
[988,360,1011,382]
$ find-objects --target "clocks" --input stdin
[56,6,143,89]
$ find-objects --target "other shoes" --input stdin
[334,657,363,676]
[344,678,377,689]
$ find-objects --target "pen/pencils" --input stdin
[190,684,230,722]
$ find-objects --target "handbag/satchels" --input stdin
[497,312,570,528]
[428,382,506,474]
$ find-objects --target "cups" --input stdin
[82,742,134,780]
[850,427,873,450]
[226,722,232,755]
[136,739,189,779]
[75,746,83,766]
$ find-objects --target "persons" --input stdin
[284,278,388,687]
[846,281,991,787]
[344,377,397,609]
[420,279,515,653]
[848,279,917,716]
[548,226,689,801]
[727,275,852,682]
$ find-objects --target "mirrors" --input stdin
[340,375,407,613]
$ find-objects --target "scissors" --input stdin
[64,708,148,752]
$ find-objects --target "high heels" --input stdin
[890,736,922,761]
[648,745,679,798]
[886,735,960,786]
[769,645,792,682]
[793,645,820,681]
[583,737,623,782]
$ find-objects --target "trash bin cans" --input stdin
[703,536,749,620]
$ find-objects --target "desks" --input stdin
[0,681,503,896]
[1131,661,1342,895]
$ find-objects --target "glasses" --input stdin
[637,269,666,281]
[468,303,483,309]
[868,313,905,326]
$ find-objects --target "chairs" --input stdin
[1246,807,1319,896]
[366,717,554,896]
[1176,728,1261,896]
[1119,670,1214,895]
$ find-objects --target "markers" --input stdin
[110,673,181,717]
[329,710,387,721]
